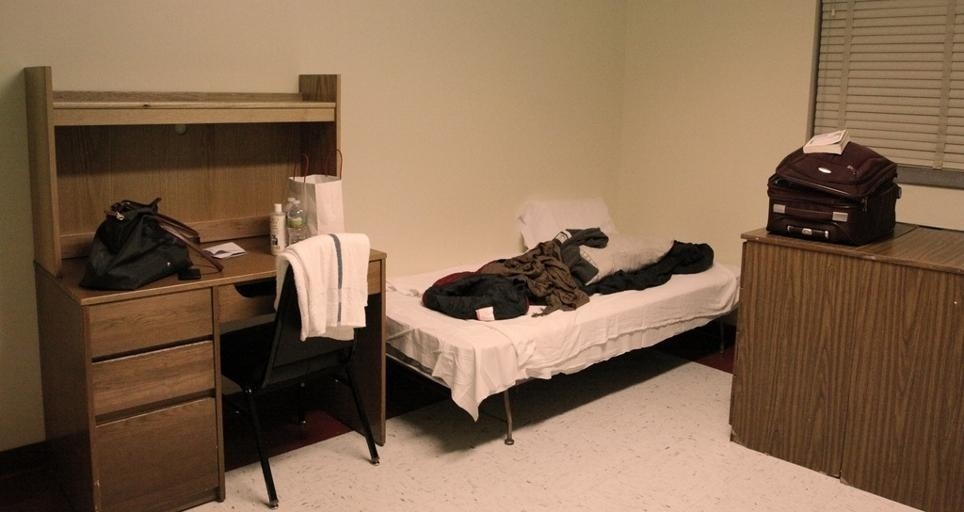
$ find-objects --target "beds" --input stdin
[385,268,738,446]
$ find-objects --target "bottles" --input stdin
[270,196,309,257]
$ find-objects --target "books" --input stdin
[801,129,852,156]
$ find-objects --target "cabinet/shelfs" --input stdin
[728,224,964,512]
[24,66,387,512]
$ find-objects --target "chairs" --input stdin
[220,234,381,507]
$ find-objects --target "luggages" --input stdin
[766,141,902,246]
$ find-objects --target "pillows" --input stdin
[515,196,671,286]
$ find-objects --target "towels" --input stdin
[269,230,372,343]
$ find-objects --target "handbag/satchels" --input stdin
[80,197,224,290]
